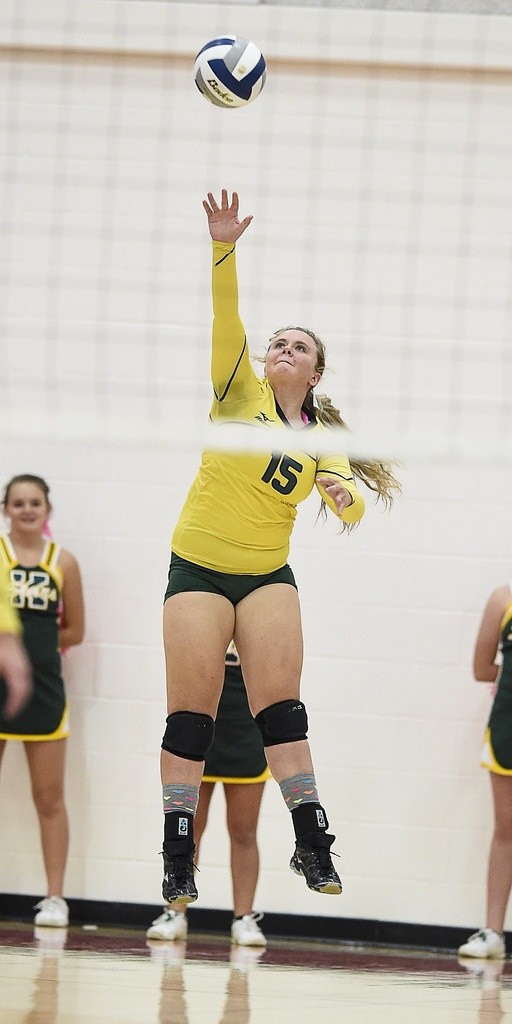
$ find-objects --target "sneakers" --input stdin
[33,925,68,941]
[145,939,187,958]
[34,895,69,927]
[146,907,188,941]
[457,956,506,975]
[289,833,342,894]
[157,838,201,903]
[230,911,267,945]
[458,928,506,958]
[230,943,266,965]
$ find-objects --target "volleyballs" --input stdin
[194,35,267,108]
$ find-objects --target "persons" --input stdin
[144,637,275,948]
[455,583,512,960]
[0,473,87,927]
[0,537,32,717]
[159,189,401,903]
